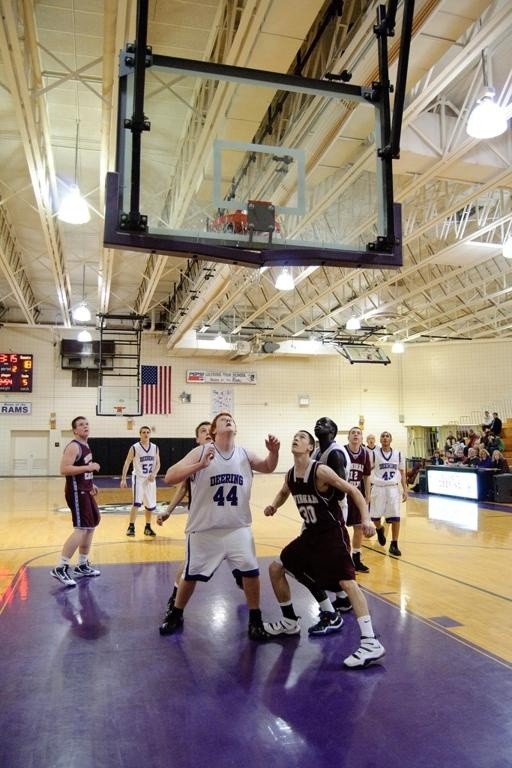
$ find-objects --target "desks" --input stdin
[404,458,434,488]
[422,463,503,505]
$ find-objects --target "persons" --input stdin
[431,411,510,474]
[120,426,161,537]
[50,416,101,586]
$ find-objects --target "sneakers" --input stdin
[50,563,76,587]
[352,552,370,573]
[343,635,387,670]
[319,595,353,612]
[375,526,387,546]
[158,596,185,635]
[126,526,135,536]
[247,622,272,643]
[389,541,401,556]
[262,616,302,636]
[307,609,344,636]
[143,526,156,536]
[73,562,101,576]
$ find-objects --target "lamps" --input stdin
[465,16,512,141]
[274,263,406,356]
[51,118,98,346]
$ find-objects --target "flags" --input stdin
[141,365,171,416]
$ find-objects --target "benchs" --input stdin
[439,417,512,473]
